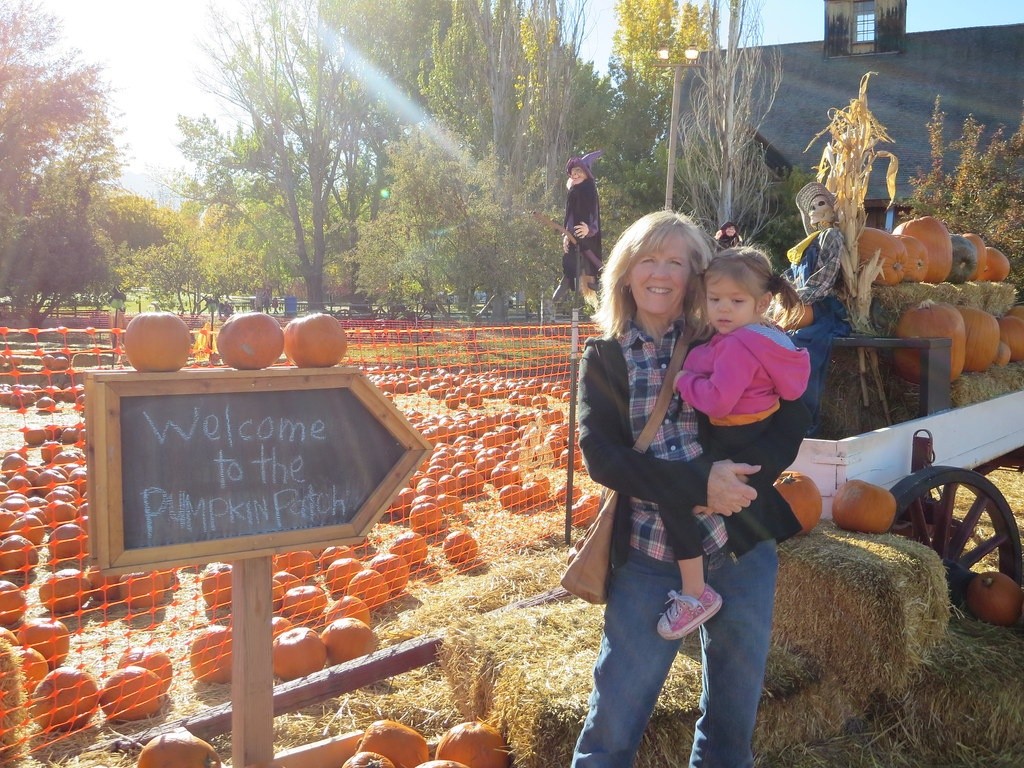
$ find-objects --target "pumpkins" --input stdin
[768,215,1024,385]
[773,470,822,535]
[967,571,1023,626]
[0,312,611,768]
[833,479,896,534]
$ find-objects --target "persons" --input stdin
[255,286,279,315]
[571,211,811,768]
[786,181,851,438]
[713,221,739,251]
[563,150,603,291]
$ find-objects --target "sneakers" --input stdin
[657,585,722,641]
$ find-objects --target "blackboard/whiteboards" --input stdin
[81,367,436,579]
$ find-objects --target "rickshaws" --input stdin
[781,334,1024,611]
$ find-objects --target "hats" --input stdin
[795,182,838,237]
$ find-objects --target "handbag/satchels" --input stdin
[561,489,618,604]
